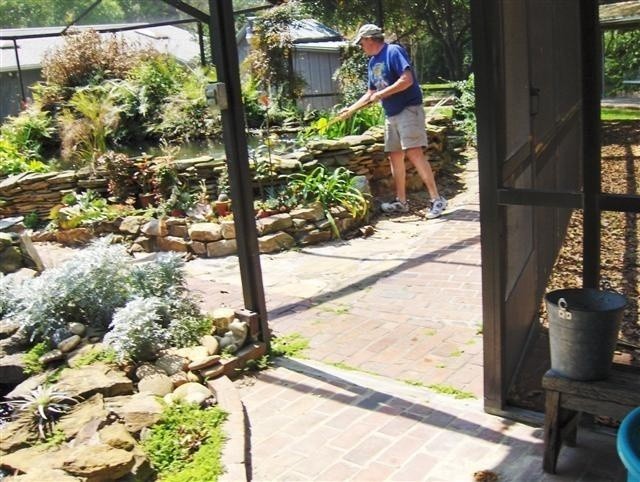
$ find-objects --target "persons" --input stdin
[339,23,448,219]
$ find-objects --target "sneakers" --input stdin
[381,197,410,213]
[425,196,448,219]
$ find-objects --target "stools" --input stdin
[539,363,640,472]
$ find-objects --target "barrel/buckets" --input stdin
[617,406,640,482]
[543,282,626,381]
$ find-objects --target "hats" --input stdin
[355,24,384,44]
